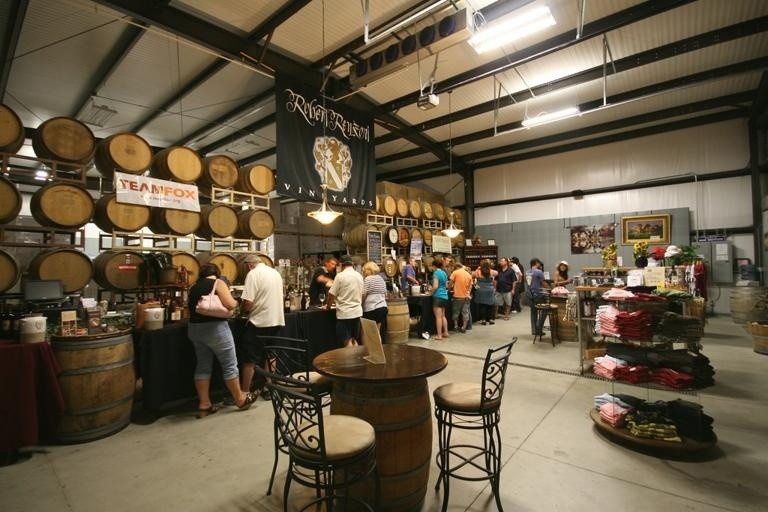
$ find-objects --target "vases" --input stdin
[666,256,681,267]
[634,255,648,268]
[654,259,665,267]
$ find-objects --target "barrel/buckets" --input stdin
[148,207,202,236]
[385,297,411,345]
[94,193,152,234]
[169,251,201,288]
[378,225,399,246]
[329,377,433,512]
[0,104,25,154]
[398,256,408,274]
[195,204,240,239]
[341,223,380,249]
[32,116,97,171]
[382,256,398,277]
[195,252,240,284]
[96,132,153,178]
[396,198,409,218]
[410,228,423,242]
[28,247,94,294]
[30,182,96,229]
[237,164,275,195]
[92,250,145,290]
[235,253,275,285]
[151,145,204,183]
[48,333,137,446]
[231,209,276,241]
[421,229,433,246]
[434,229,465,248]
[445,206,464,225]
[0,248,21,293]
[378,195,397,216]
[342,192,381,216]
[396,226,411,247]
[201,155,240,188]
[0,174,23,225]
[432,202,446,221]
[419,201,434,219]
[405,199,421,219]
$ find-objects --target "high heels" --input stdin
[239,388,261,412]
[199,405,218,419]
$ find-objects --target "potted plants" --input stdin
[678,244,700,264]
[607,258,618,268]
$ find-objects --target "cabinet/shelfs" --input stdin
[132,303,336,421]
[0,338,48,458]
[407,289,481,338]
[0,152,96,298]
[395,218,418,289]
[443,223,461,256]
[191,184,270,298]
[419,219,443,282]
[691,241,733,284]
[260,202,362,266]
[582,266,690,305]
[97,167,195,311]
[460,245,498,271]
[575,285,688,376]
[346,212,393,287]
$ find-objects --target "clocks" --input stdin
[385,226,399,247]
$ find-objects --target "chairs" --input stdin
[530,288,561,347]
[254,366,380,512]
[255,335,332,505]
[433,336,518,512]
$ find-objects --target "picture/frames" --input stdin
[620,213,671,245]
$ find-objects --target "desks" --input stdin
[549,293,577,342]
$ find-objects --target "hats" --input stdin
[559,261,567,268]
[240,253,260,264]
[339,255,353,265]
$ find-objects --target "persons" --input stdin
[402,257,419,297]
[362,261,388,344]
[512,257,525,312]
[320,255,364,347]
[525,258,549,335]
[494,256,518,321]
[448,262,473,333]
[224,254,285,406]
[471,259,499,325]
[308,256,339,305]
[507,256,523,313]
[187,263,262,418]
[553,261,570,289]
[430,259,449,340]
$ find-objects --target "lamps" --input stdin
[306,183,343,225]
[467,0,557,55]
[441,211,464,238]
[521,106,581,128]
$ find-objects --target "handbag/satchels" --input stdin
[194,281,236,318]
[525,275,545,304]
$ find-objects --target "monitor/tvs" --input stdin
[410,237,423,261]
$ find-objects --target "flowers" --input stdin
[664,245,685,258]
[599,242,621,268]
[631,240,651,263]
[649,246,667,261]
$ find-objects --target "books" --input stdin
[626,266,666,286]
[359,317,387,364]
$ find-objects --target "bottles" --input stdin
[301,289,309,310]
[12,302,25,339]
[284,290,291,314]
[405,281,409,296]
[178,265,188,285]
[1,304,16,338]
[583,301,592,317]
[668,264,677,283]
[168,299,176,323]
[166,299,170,320]
